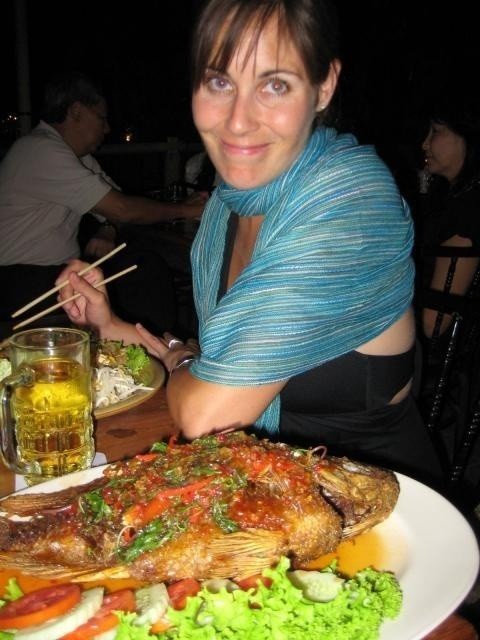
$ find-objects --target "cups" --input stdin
[417,170,429,193]
[3,328,93,486]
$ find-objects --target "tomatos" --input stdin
[1,573,271,639]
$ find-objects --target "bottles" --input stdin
[199,190,209,205]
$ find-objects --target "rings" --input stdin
[168,336,184,349]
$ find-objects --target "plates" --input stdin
[2,331,168,427]
[1,442,480,635]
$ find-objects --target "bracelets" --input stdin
[168,356,195,376]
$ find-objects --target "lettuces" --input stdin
[111,555,402,639]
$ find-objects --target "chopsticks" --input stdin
[6,242,140,331]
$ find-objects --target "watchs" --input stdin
[100,224,117,236]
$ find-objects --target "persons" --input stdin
[421,121,480,339]
[0,85,208,264]
[54,0,445,495]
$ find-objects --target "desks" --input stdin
[80,182,480,410]
